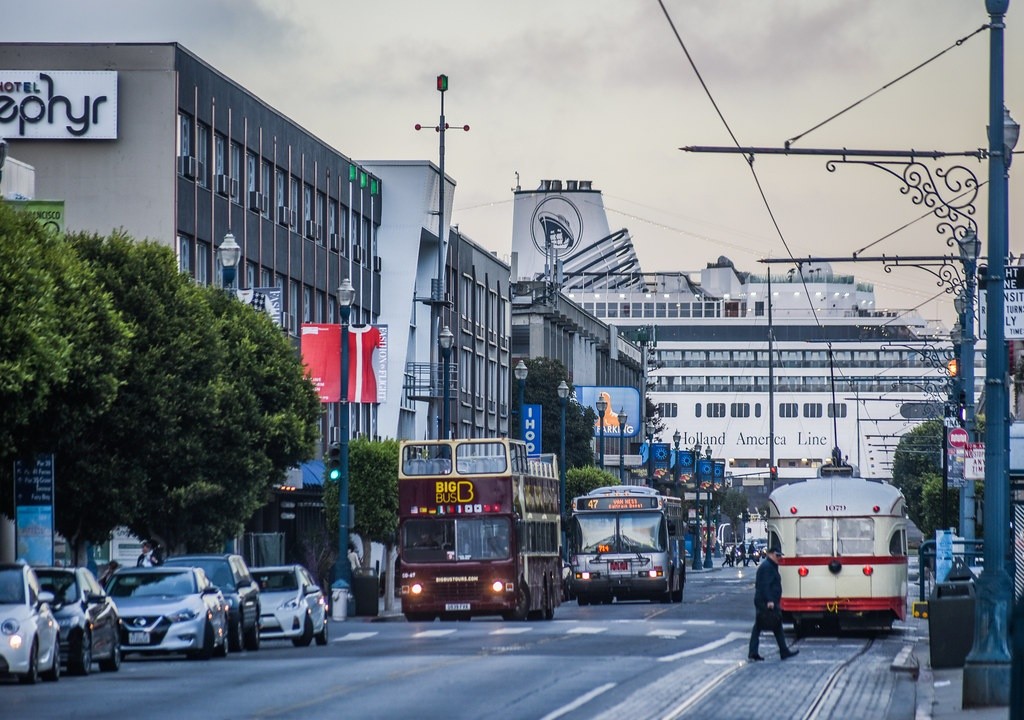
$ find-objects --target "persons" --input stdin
[914,541,936,586]
[747,547,800,661]
[348,543,362,575]
[99,560,119,588]
[395,548,402,589]
[136,542,163,567]
[722,540,759,568]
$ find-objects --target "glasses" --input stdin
[776,554,781,557]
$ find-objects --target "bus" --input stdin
[398,440,562,620]
[765,341,910,635]
[568,485,686,603]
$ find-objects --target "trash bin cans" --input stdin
[352,567,379,617]
[927,582,975,670]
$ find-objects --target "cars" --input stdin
[0,558,60,681]
[104,568,231,659]
[251,566,332,647]
[37,568,124,671]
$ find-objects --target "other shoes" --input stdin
[748,655,765,661]
[781,650,799,659]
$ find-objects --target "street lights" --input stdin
[617,407,628,485]
[596,392,608,471]
[704,446,713,567]
[335,277,356,588]
[692,443,702,569]
[440,326,454,459]
[557,380,569,530]
[220,236,241,554]
[957,225,982,567]
[515,359,529,442]
[674,431,681,496]
[646,421,655,487]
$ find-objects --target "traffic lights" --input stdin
[329,442,341,486]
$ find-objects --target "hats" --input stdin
[768,546,784,556]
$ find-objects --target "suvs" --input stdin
[161,555,261,652]
